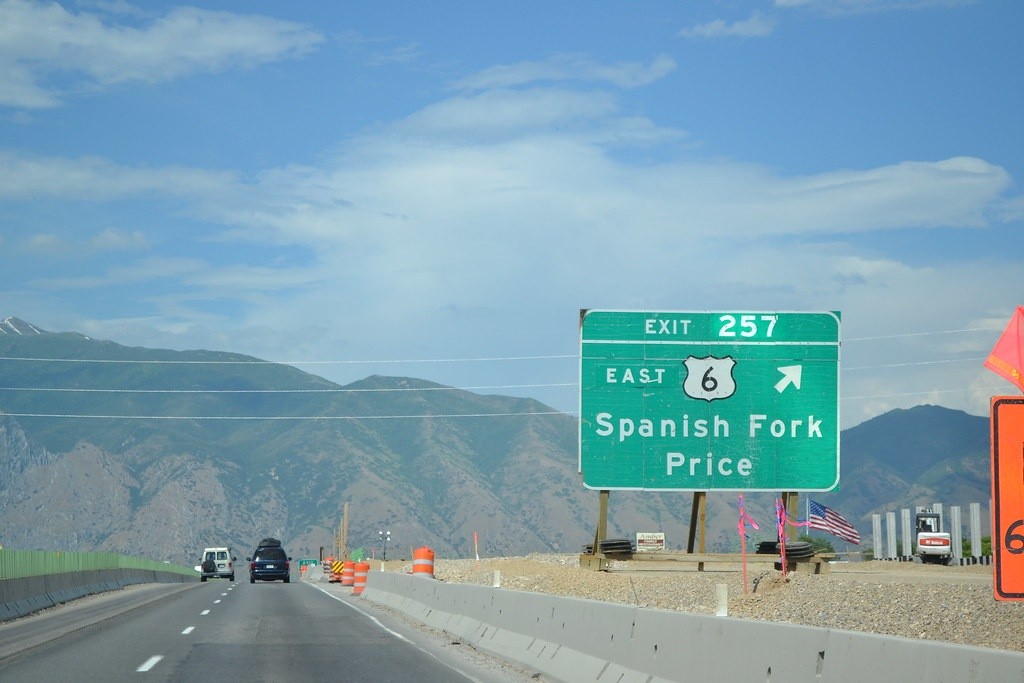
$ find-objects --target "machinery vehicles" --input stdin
[915,511,953,565]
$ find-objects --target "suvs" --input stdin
[246,546,293,583]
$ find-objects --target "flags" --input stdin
[809,499,860,545]
[984,306,1024,394]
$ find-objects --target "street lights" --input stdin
[378,530,391,561]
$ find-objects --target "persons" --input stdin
[921,521,925,531]
[221,554,224,557]
[210,554,213,558]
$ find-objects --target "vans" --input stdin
[198,547,237,582]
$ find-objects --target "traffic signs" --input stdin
[578,307,842,493]
